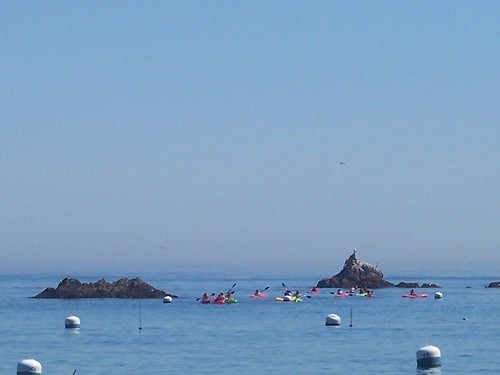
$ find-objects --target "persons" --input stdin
[296,291,299,294]
[360,287,364,294]
[350,286,355,293]
[411,289,414,295]
[312,287,317,291]
[203,292,231,300]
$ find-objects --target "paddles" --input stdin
[230,291,235,294]
[196,293,215,301]
[329,292,352,296]
[282,283,288,290]
[303,295,311,298]
[228,283,236,292]
[260,287,269,292]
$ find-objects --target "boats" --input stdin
[335,292,375,298]
[250,293,268,298]
[276,290,320,302]
[402,293,428,298]
[200,298,237,304]
